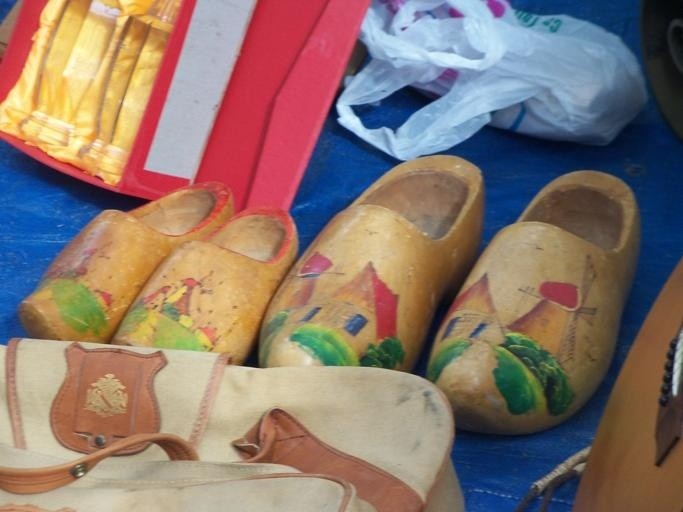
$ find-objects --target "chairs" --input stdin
[261,154,485,369]
[111,206,300,366]
[429,170,641,434]
[17,181,232,342]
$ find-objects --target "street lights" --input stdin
[0,340,465,512]
